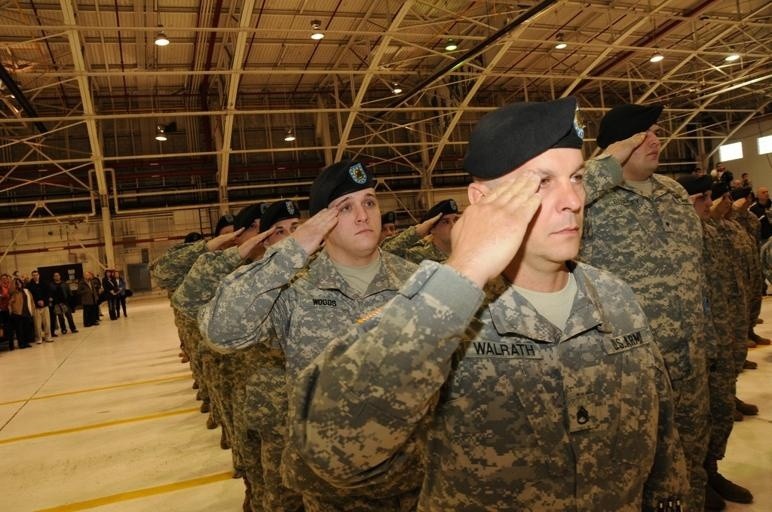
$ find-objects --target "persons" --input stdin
[146,96,771,510]
[0,268,133,354]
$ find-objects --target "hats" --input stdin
[463,96,583,179]
[260,200,301,233]
[597,102,662,150]
[184,232,201,243]
[429,199,458,219]
[215,215,235,237]
[382,212,395,225]
[233,203,270,231]
[310,162,374,217]
[676,175,752,201]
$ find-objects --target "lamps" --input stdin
[444,38,458,51]
[154,25,169,47]
[392,81,404,98]
[554,10,567,49]
[309,23,325,40]
[151,120,300,144]
[724,47,740,61]
[650,0,664,63]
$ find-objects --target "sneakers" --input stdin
[36,329,78,343]
[742,318,771,369]
[179,352,189,363]
[84,312,127,327]
[735,396,758,421]
[705,472,753,512]
[193,383,243,478]
